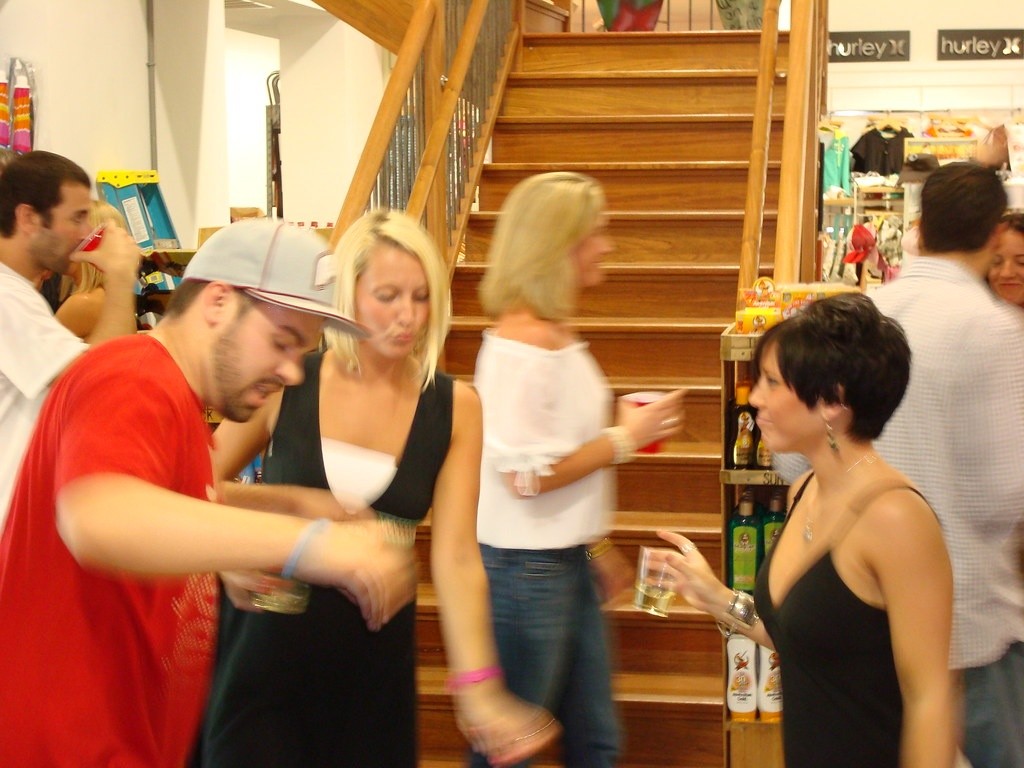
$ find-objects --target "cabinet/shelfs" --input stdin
[719,323,792,768]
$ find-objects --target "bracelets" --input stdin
[603,422,636,463]
[442,664,505,692]
[585,538,616,560]
[281,518,330,582]
[716,587,760,636]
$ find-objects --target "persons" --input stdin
[0,217,417,768]
[0,152,143,531]
[467,170,684,768]
[638,159,1024,768]
[190,205,564,768]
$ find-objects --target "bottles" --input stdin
[729,381,774,471]
[728,495,784,592]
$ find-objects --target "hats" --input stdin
[183,215,379,341]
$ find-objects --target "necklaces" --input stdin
[803,457,865,541]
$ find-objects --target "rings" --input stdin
[677,542,699,553]
[661,416,680,429]
[512,716,556,742]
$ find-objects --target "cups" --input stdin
[624,394,665,453]
[72,226,105,254]
[631,543,682,619]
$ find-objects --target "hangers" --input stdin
[818,107,1024,141]
[821,201,903,280]
